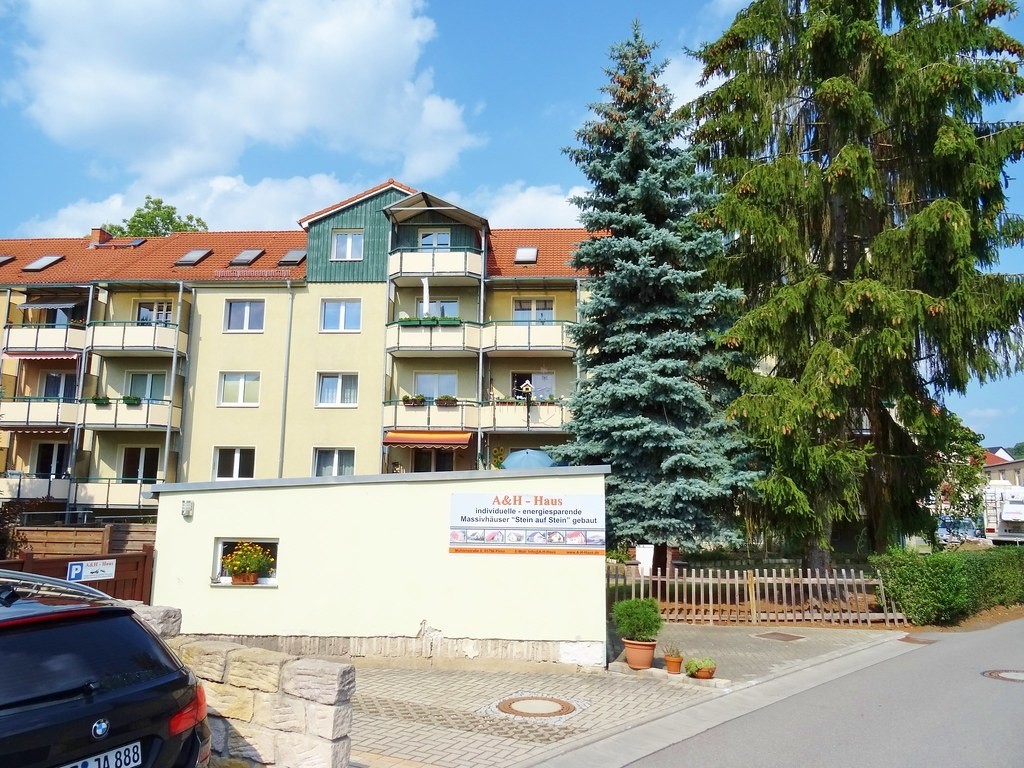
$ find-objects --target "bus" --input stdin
[975,479,1024,542]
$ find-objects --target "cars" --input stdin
[936,515,980,544]
[0,569,213,768]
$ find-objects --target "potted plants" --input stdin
[662,640,683,674]
[91,394,109,404]
[435,394,457,406]
[438,315,462,326]
[420,315,439,326]
[610,596,665,669]
[496,394,555,406]
[398,317,421,326]
[123,394,142,405]
[684,656,716,679]
[402,394,426,405]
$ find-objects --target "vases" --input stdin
[69,325,85,330]
[231,572,258,585]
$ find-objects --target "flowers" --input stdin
[221,540,275,576]
[69,318,85,324]
